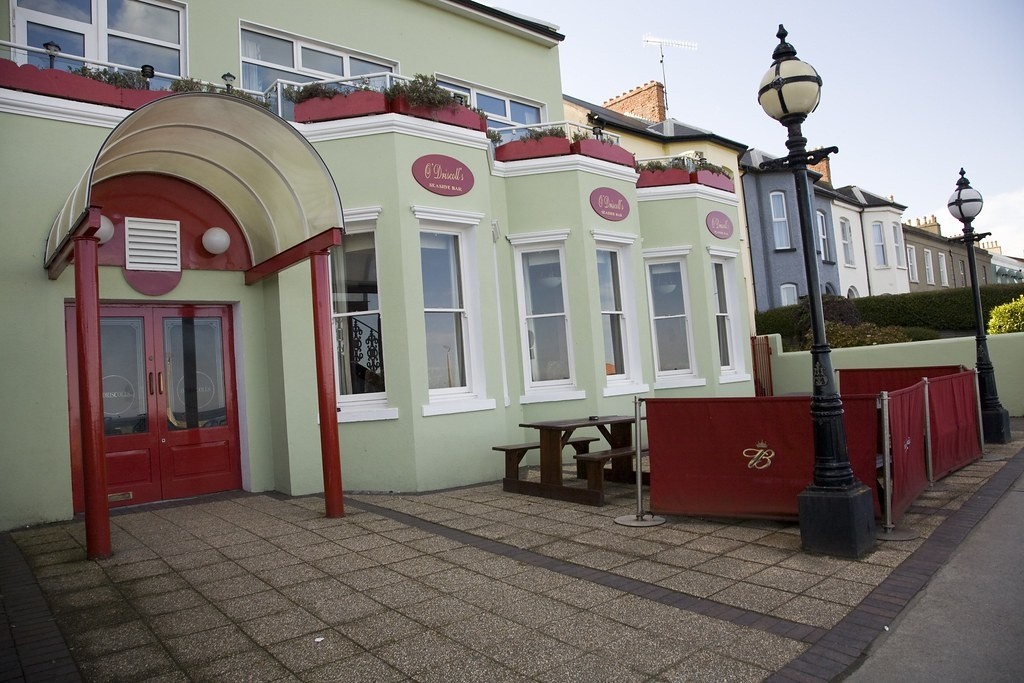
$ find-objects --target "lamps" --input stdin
[699,157,707,167]
[202,226,231,255]
[42,40,61,69]
[588,111,600,122]
[453,95,464,106]
[93,213,115,246]
[222,71,236,95]
[593,126,602,141]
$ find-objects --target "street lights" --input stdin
[946,167,1012,445]
[757,21,880,561]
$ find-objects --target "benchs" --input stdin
[492,437,650,508]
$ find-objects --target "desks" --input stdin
[519,414,647,485]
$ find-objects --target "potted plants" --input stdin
[0,58,268,113]
[489,123,634,167]
[636,153,736,194]
[281,69,490,132]
